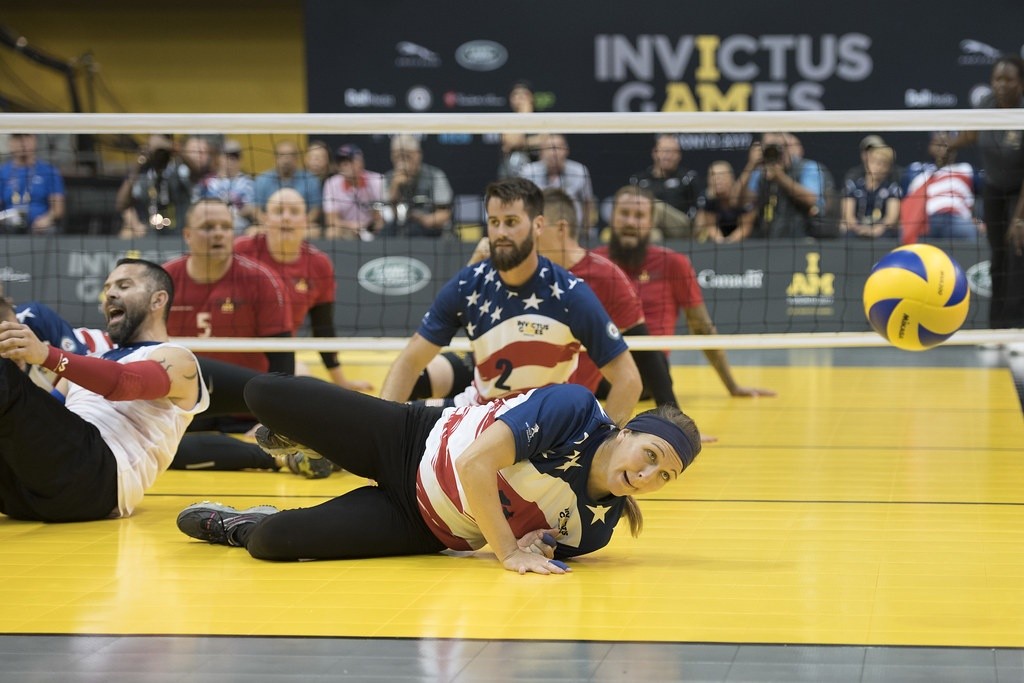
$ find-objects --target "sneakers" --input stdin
[175,501,279,547]
[254,422,323,461]
[285,450,340,479]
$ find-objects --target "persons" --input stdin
[118,55,1024,329]
[0,258,210,523]
[231,188,374,391]
[176,374,701,577]
[588,186,775,402]
[0,296,342,479]
[0,133,65,238]
[379,176,643,428]
[412,187,717,443]
[160,198,295,435]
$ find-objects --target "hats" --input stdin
[336,141,362,162]
[860,134,887,150]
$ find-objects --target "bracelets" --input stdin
[1012,217,1024,226]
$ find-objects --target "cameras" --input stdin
[761,144,780,162]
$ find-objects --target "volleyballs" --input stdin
[861,242,972,353]
